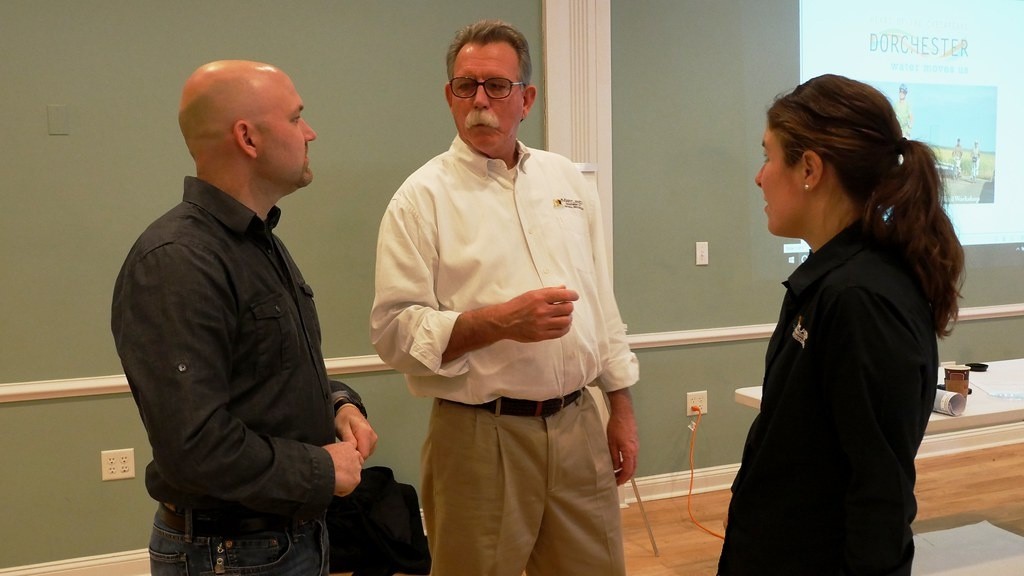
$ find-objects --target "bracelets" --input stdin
[334,397,368,418]
[335,404,360,414]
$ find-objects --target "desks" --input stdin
[734,358,1023,438]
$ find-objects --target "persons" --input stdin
[368,18,640,576]
[715,73,966,575]
[892,82,914,139]
[970,141,982,182]
[951,137,964,180]
[108,58,378,576]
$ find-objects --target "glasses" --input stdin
[449,76,529,100]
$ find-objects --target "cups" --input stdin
[943,365,971,401]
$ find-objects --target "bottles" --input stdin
[952,139,962,179]
[969,142,981,183]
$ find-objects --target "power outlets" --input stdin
[101,448,136,481]
[686,390,708,417]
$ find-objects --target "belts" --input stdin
[442,386,585,417]
[158,507,321,537]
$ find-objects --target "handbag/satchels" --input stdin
[325,466,431,576]
